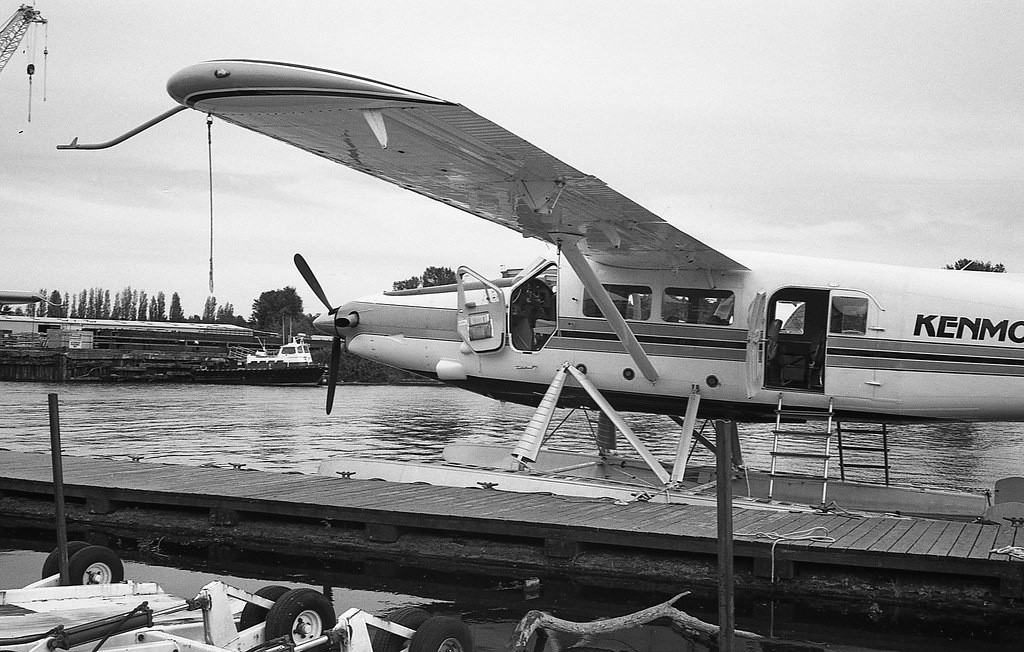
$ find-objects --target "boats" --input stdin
[192,331,328,387]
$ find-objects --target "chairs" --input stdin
[667,314,827,368]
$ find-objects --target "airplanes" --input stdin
[56,59,1024,525]
